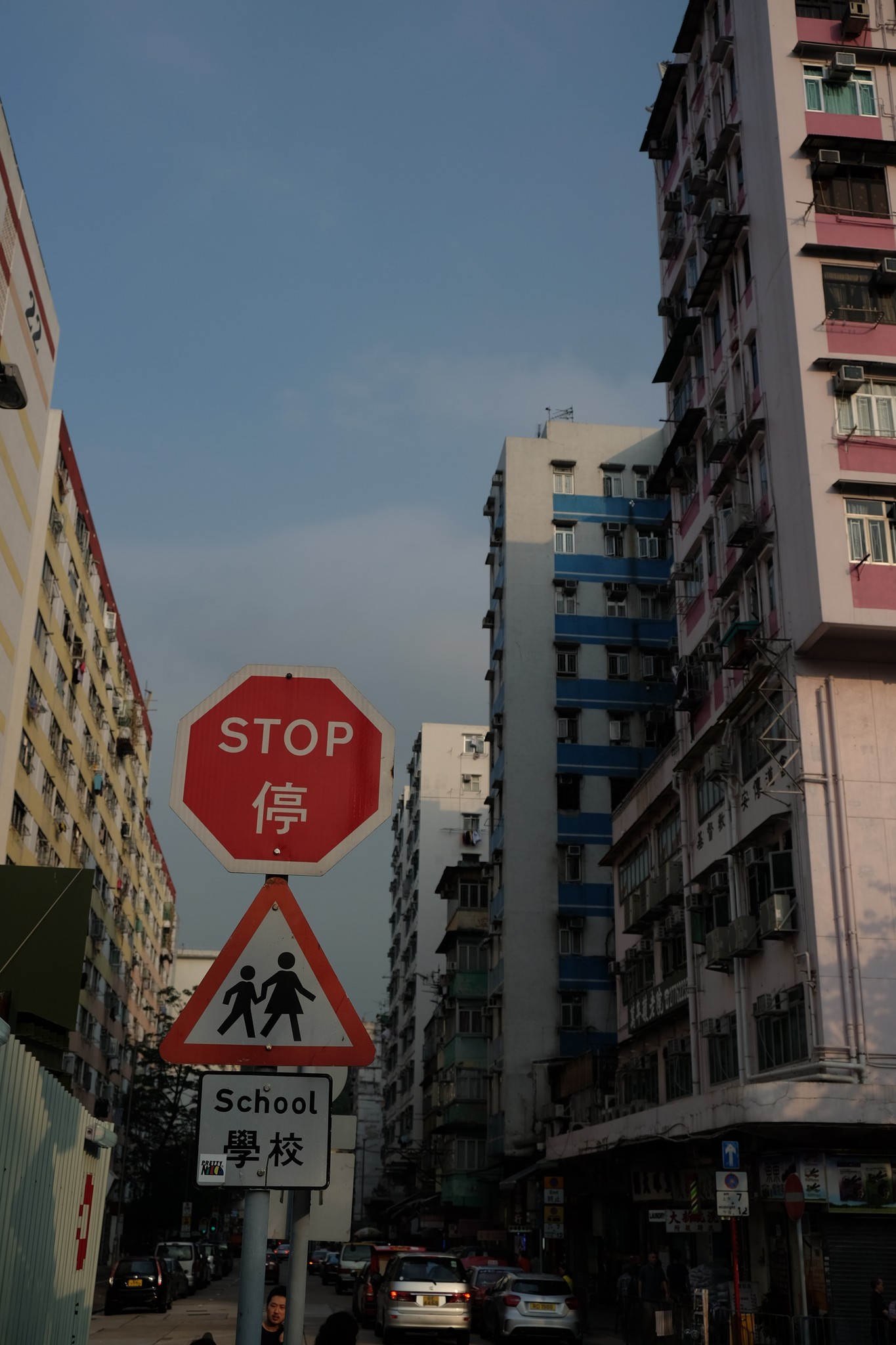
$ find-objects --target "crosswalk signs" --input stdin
[159,877,377,1068]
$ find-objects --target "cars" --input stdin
[308,1241,525,1338]
[374,1252,476,1345]
[104,1256,173,1314]
[488,1272,580,1344]
[149,1242,235,1311]
[274,1244,291,1260]
[265,1252,281,1284]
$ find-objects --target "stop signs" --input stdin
[167,663,396,876]
[784,1173,806,1222]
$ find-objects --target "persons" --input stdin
[558,1266,576,1294]
[666,1251,692,1333]
[614,1267,637,1328]
[312,1310,358,1345]
[869,1277,889,1345]
[260,1288,286,1345]
[518,1250,531,1273]
[190,1332,217,1344]
[637,1252,671,1334]
